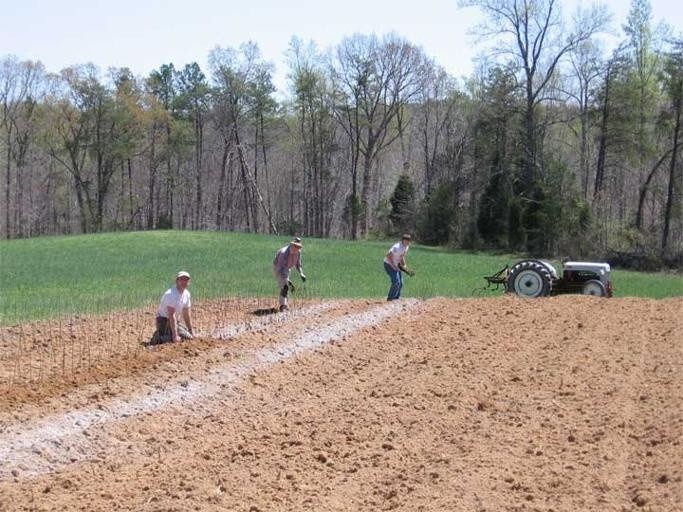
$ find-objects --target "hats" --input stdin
[176,271,190,279]
[289,238,302,248]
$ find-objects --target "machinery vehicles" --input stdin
[472,256,614,297]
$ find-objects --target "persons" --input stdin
[149,271,195,345]
[271,237,306,312]
[384,234,415,302]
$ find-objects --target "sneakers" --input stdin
[148,330,161,346]
